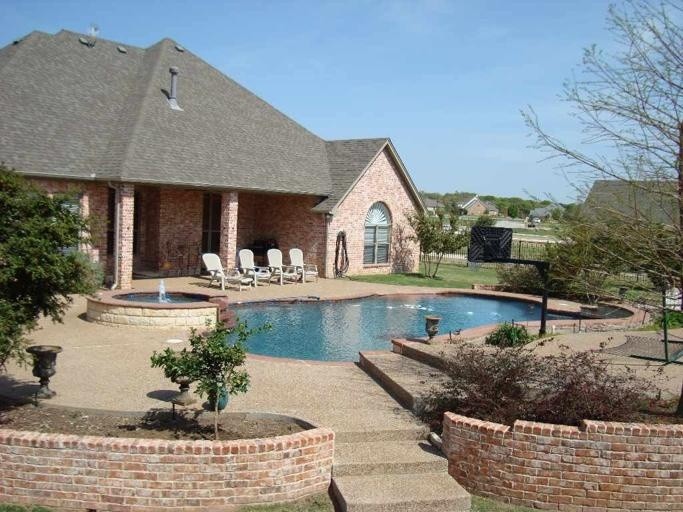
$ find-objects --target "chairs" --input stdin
[200,246,319,293]
